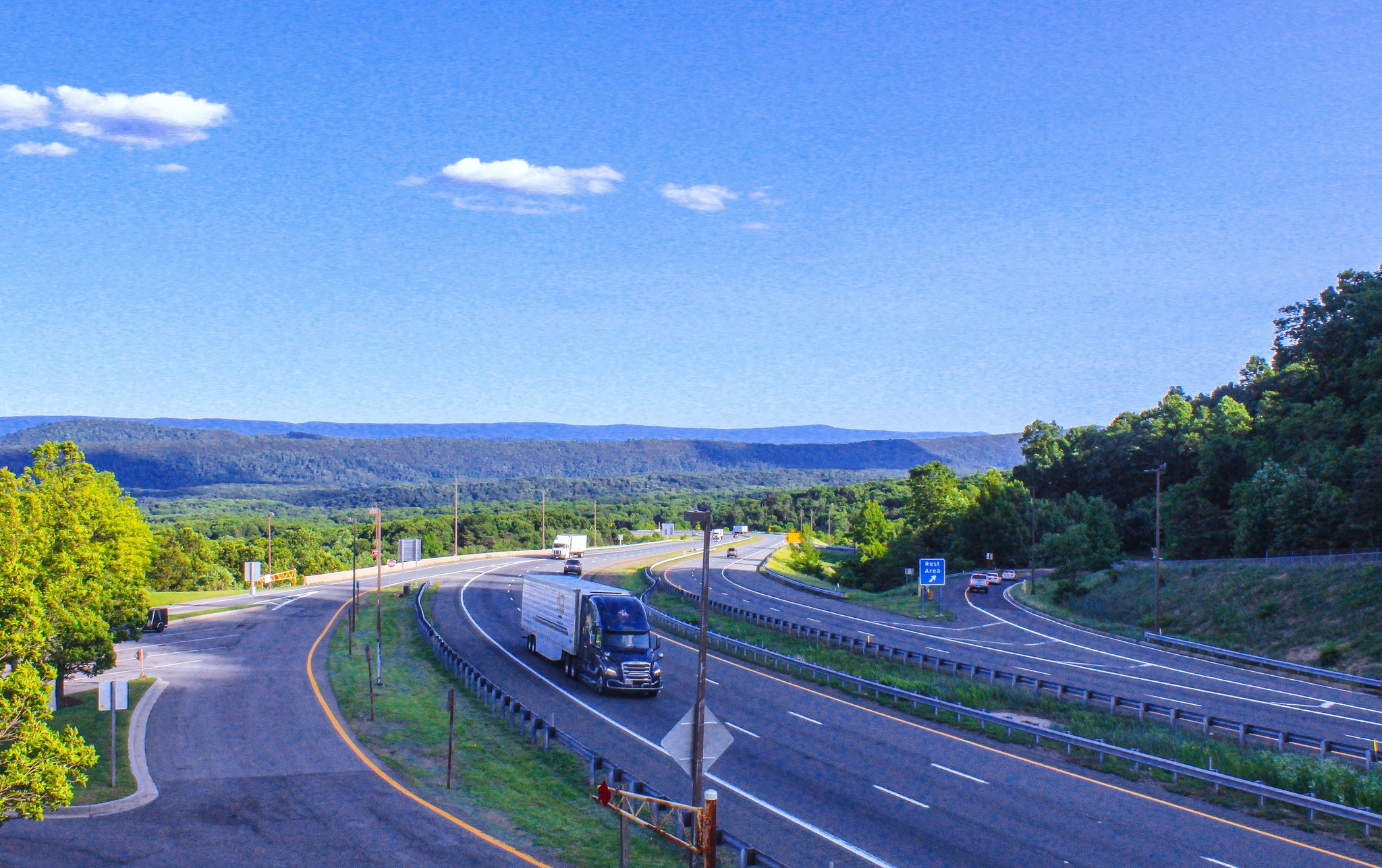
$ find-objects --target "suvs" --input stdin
[969,573,989,594]
[563,559,583,575]
[727,548,738,558]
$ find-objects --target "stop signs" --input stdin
[387,559,396,568]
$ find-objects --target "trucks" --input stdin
[733,525,748,534]
[712,528,724,542]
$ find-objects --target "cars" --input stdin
[733,533,738,538]
[986,572,1001,585]
[692,532,698,536]
[1002,570,1019,582]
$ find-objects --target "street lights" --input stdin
[268,512,275,588]
[683,510,712,868]
[347,518,356,633]
[1144,463,1167,632]
[367,508,383,686]
[539,489,545,549]
[800,508,830,535]
[1027,485,1040,595]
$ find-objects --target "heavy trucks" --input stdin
[550,533,587,559]
[520,573,664,698]
